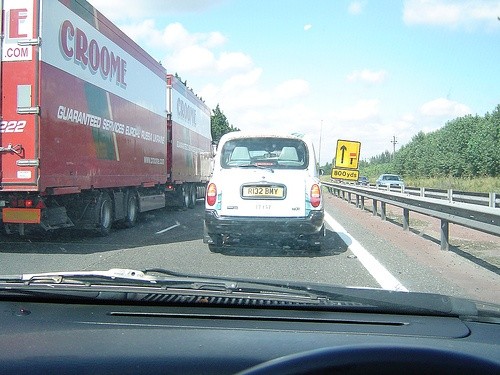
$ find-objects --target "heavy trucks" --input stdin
[0,0,216,241]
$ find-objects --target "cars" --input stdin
[331,176,370,186]
[203,130,325,253]
[376,174,405,189]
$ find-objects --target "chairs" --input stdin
[278,147,301,167]
[227,147,250,166]
